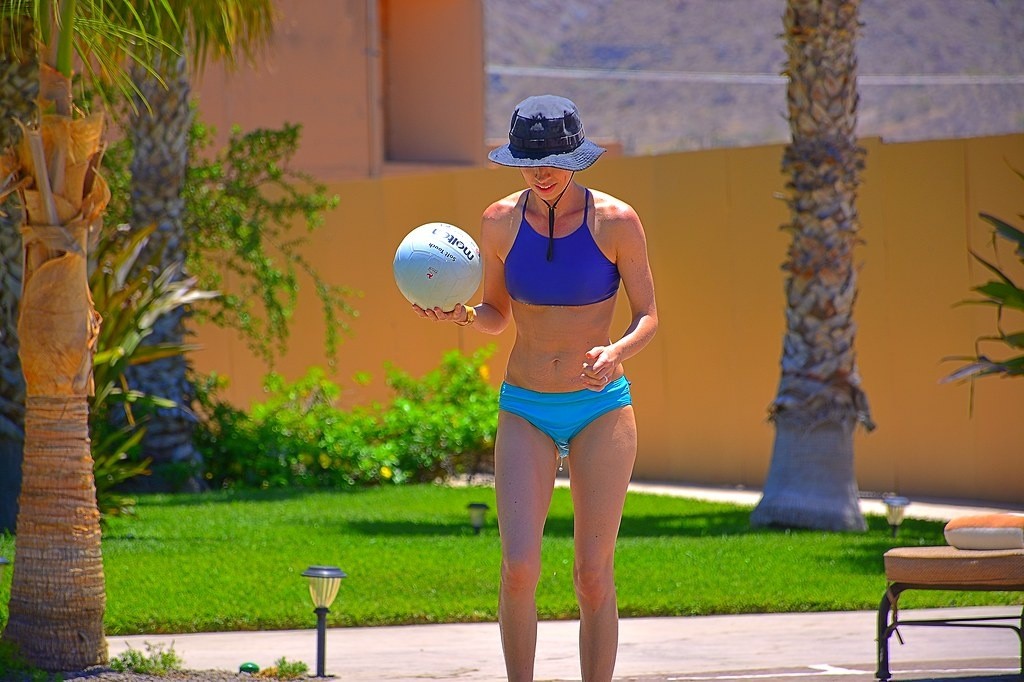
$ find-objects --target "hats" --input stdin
[488,94,607,171]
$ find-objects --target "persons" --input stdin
[410,92,658,682]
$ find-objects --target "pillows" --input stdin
[942,512,1024,550]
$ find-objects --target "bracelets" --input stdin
[453,304,474,327]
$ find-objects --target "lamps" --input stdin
[299,564,347,614]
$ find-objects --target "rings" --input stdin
[604,376,609,383]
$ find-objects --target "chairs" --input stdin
[874,546,1024,682]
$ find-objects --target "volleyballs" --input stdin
[393,222,483,313]
